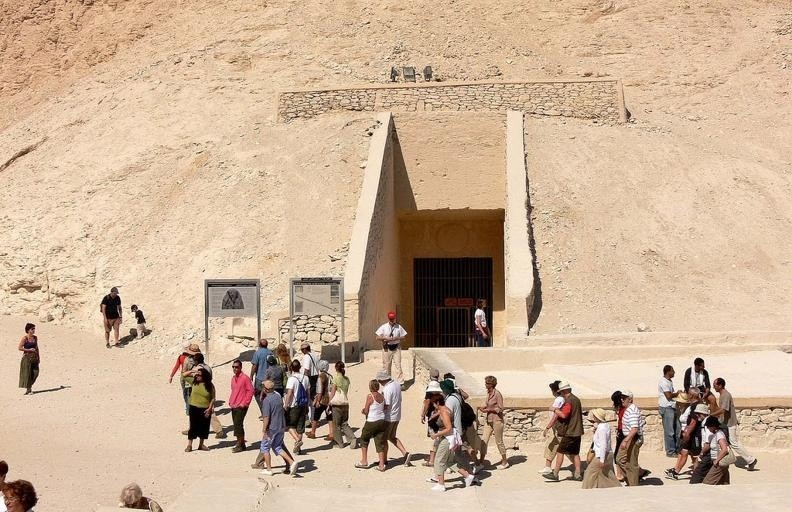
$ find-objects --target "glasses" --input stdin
[196,374,202,377]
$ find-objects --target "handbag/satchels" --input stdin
[717,449,735,468]
[480,324,491,340]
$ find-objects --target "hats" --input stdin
[265,353,278,365]
[260,379,276,390]
[549,379,575,392]
[704,415,722,427]
[619,390,634,400]
[591,407,609,422]
[182,341,202,355]
[387,310,397,320]
[297,341,310,351]
[374,368,393,382]
[425,380,443,395]
[693,403,711,415]
[671,390,691,406]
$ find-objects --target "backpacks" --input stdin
[293,376,310,408]
[454,395,477,430]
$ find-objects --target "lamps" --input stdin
[389,64,434,85]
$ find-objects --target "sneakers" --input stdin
[354,459,372,470]
[292,437,305,455]
[472,461,486,475]
[463,472,475,486]
[183,444,211,455]
[286,460,298,476]
[494,461,512,470]
[430,484,447,492]
[323,432,344,448]
[231,440,247,453]
[305,431,317,438]
[537,465,560,482]
[663,468,680,481]
[566,472,582,481]
[348,437,360,449]
[403,451,414,468]
[747,459,757,471]
[249,460,275,476]
[374,464,388,472]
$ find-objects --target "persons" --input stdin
[101,287,124,349]
[130,303,150,340]
[17,323,40,396]
[117,481,165,512]
[473,296,493,347]
[0,460,10,511]
[1,478,40,512]
[374,310,408,391]
